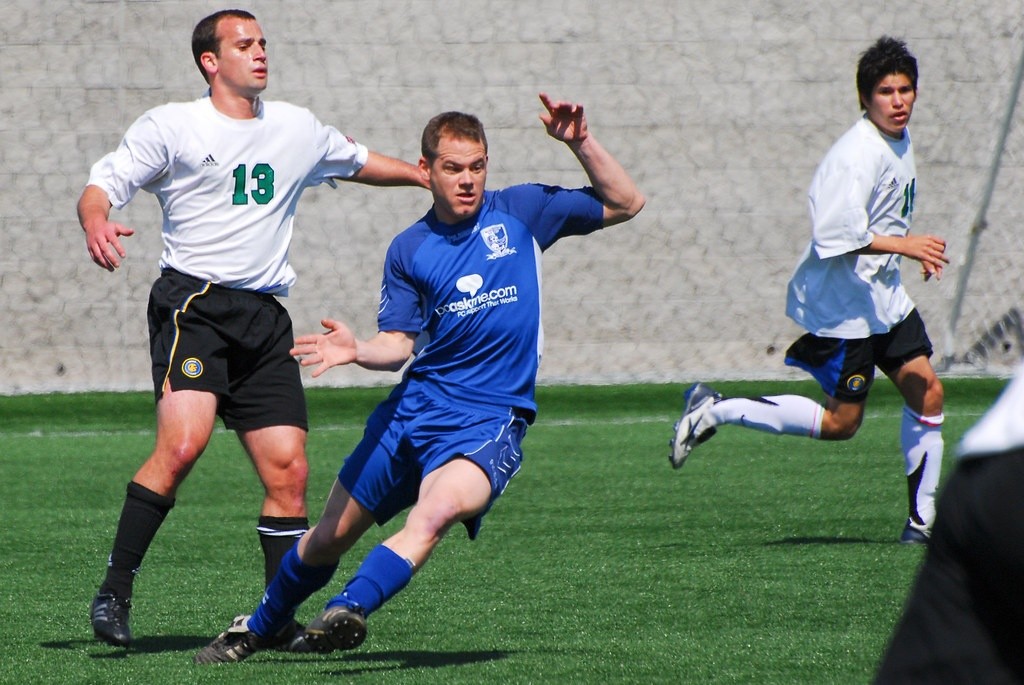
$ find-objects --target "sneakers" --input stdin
[193,616,260,662]
[900,518,933,544]
[91,591,131,646]
[669,383,726,470]
[304,606,367,655]
[267,623,315,654]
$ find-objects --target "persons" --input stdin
[76,9,432,647]
[191,92,647,665]
[870,368,1024,685]
[668,35,944,545]
